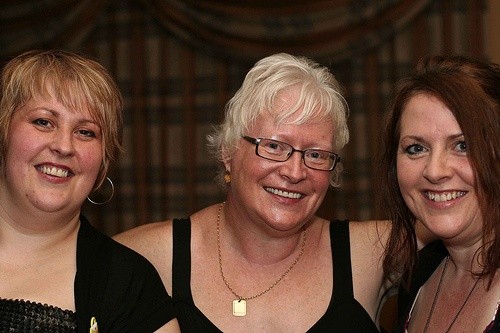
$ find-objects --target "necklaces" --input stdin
[423,254,487,333]
[217,200,307,318]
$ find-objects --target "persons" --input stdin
[381,57,500,333]
[112,53,440,333]
[0,50,180,333]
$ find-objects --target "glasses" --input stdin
[240,133,341,171]
